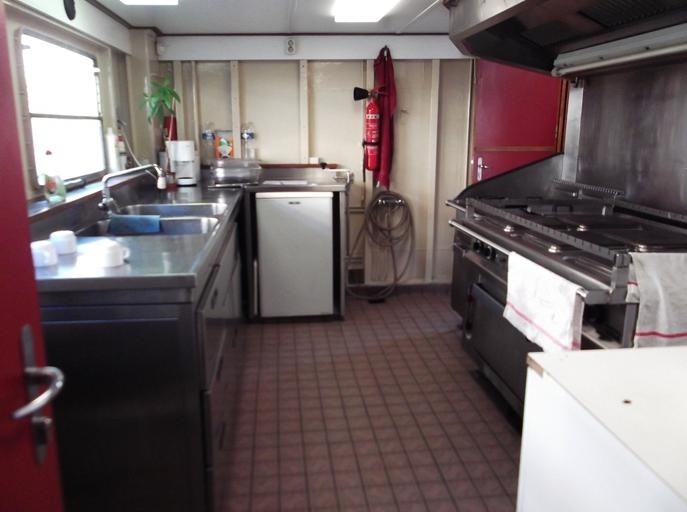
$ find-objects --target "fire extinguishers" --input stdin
[353,86,379,171]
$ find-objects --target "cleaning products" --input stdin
[42,147,67,206]
[105,127,121,172]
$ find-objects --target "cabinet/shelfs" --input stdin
[33,221,250,512]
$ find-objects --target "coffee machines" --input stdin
[165,139,199,186]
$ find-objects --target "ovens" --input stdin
[465,251,630,423]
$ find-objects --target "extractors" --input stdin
[441,0,687,88]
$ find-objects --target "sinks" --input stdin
[118,203,229,220]
[72,216,220,237]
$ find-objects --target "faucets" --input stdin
[98,162,168,216]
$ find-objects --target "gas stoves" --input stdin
[464,192,602,261]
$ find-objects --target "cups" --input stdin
[49,229,77,253]
[30,240,58,269]
